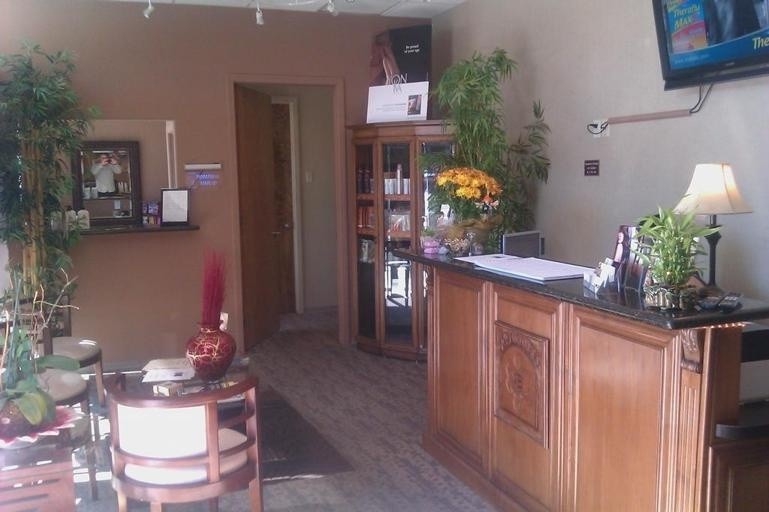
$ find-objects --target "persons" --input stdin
[366,32,401,85]
[90,153,123,197]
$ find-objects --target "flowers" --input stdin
[435,164,505,209]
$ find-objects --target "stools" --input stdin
[37,335,106,409]
[34,368,100,503]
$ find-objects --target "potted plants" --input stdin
[621,200,725,310]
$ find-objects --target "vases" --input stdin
[183,320,237,385]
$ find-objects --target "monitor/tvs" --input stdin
[653,0,769,94]
[501,228,544,258]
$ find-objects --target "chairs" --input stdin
[102,370,264,511]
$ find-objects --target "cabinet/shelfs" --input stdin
[343,121,458,363]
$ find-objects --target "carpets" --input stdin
[256,391,355,481]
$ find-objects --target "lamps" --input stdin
[671,161,755,310]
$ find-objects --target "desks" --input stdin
[138,359,255,414]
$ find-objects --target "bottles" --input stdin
[48,206,91,232]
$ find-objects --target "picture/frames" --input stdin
[71,139,143,227]
